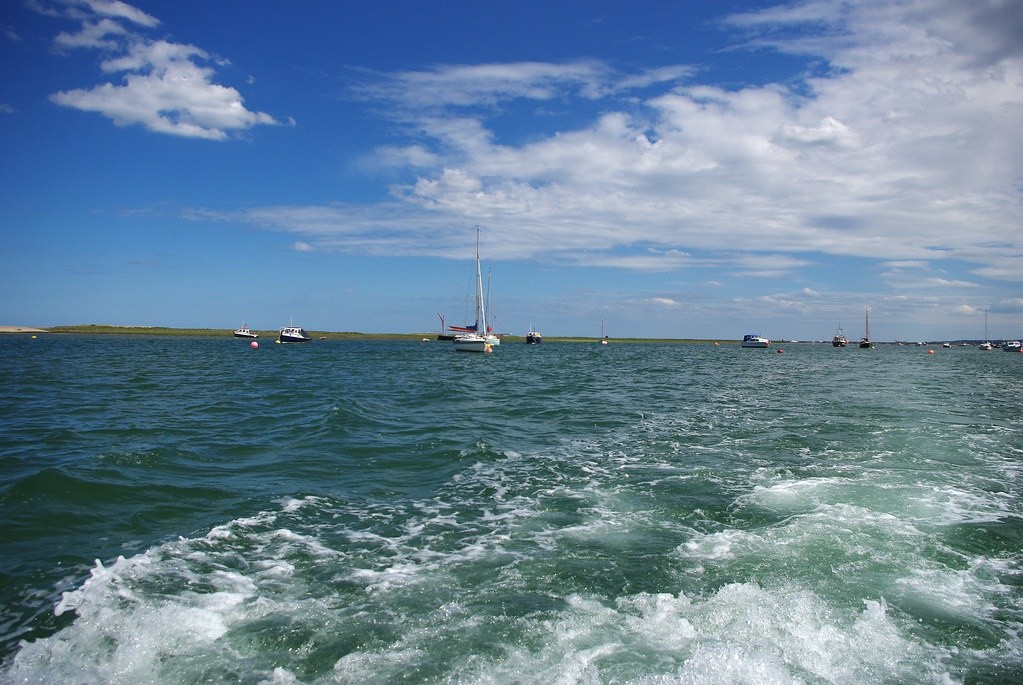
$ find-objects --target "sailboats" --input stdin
[860,306,876,348]
[600,320,608,346]
[832,322,848,347]
[454,258,492,353]
[978,310,991,350]
[446,275,511,336]
[436,224,480,340]
[453,266,502,346]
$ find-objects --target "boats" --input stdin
[1002,341,1021,352]
[279,314,311,342]
[992,344,1002,348]
[741,334,769,349]
[915,341,927,346]
[526,321,541,344]
[790,339,798,343]
[233,320,259,338]
[942,342,951,348]
[973,342,977,347]
[958,342,967,346]
